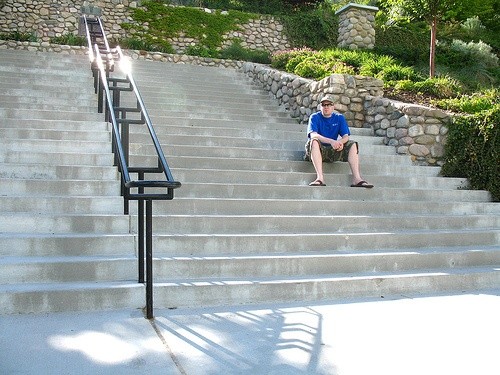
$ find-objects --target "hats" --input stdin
[320,97,333,102]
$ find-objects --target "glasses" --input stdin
[321,102,334,106]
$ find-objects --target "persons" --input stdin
[303,95,374,187]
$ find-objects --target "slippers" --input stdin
[309,180,326,186]
[350,182,374,188]
